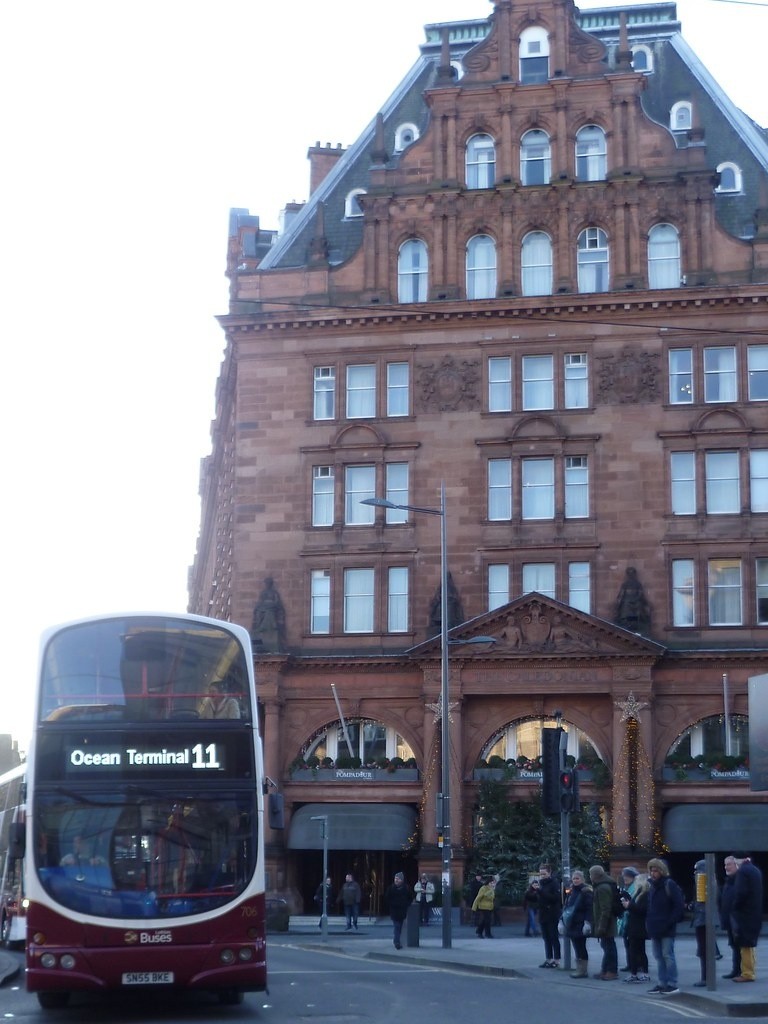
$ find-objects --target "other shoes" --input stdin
[731,973,755,983]
[418,923,429,927]
[693,981,707,987]
[477,934,494,939]
[525,932,542,937]
[318,927,323,931]
[620,967,633,972]
[344,925,358,933]
[722,972,740,979]
[594,970,618,981]
[393,938,403,950]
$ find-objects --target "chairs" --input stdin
[170,708,199,720]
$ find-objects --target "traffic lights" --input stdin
[561,770,582,816]
[538,726,564,818]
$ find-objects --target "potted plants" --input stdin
[593,758,602,769]
[322,757,333,770]
[406,758,416,769]
[742,752,750,767]
[669,753,683,767]
[714,753,723,769]
[578,755,592,770]
[391,758,403,770]
[306,757,320,769]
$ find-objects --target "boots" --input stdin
[569,958,589,978]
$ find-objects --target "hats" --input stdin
[395,872,404,881]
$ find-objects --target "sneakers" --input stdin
[621,974,639,985]
[647,984,662,994]
[539,961,560,969]
[661,985,679,994]
[633,975,650,984]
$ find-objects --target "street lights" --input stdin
[310,814,332,942]
[359,495,462,951]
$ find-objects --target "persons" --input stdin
[468,874,501,938]
[589,865,619,980]
[522,880,541,937]
[385,872,412,949]
[619,867,651,983]
[722,851,762,982]
[316,877,332,929]
[685,860,723,986]
[59,835,105,866]
[336,874,361,931]
[491,616,523,650]
[431,571,460,625]
[537,864,562,969]
[254,578,286,630]
[550,615,590,652]
[114,834,137,858]
[198,681,240,719]
[646,859,681,995]
[414,873,435,926]
[618,568,647,625]
[558,871,593,979]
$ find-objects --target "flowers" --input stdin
[515,759,540,772]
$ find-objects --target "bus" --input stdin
[22,610,269,1015]
[0,761,30,948]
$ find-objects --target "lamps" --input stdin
[503,289,513,296]
[439,292,447,300]
[559,173,568,179]
[502,177,512,184]
[625,282,635,288]
[558,286,567,292]
[501,75,510,81]
[554,70,562,76]
[370,297,379,304]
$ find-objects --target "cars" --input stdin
[267,895,291,933]
